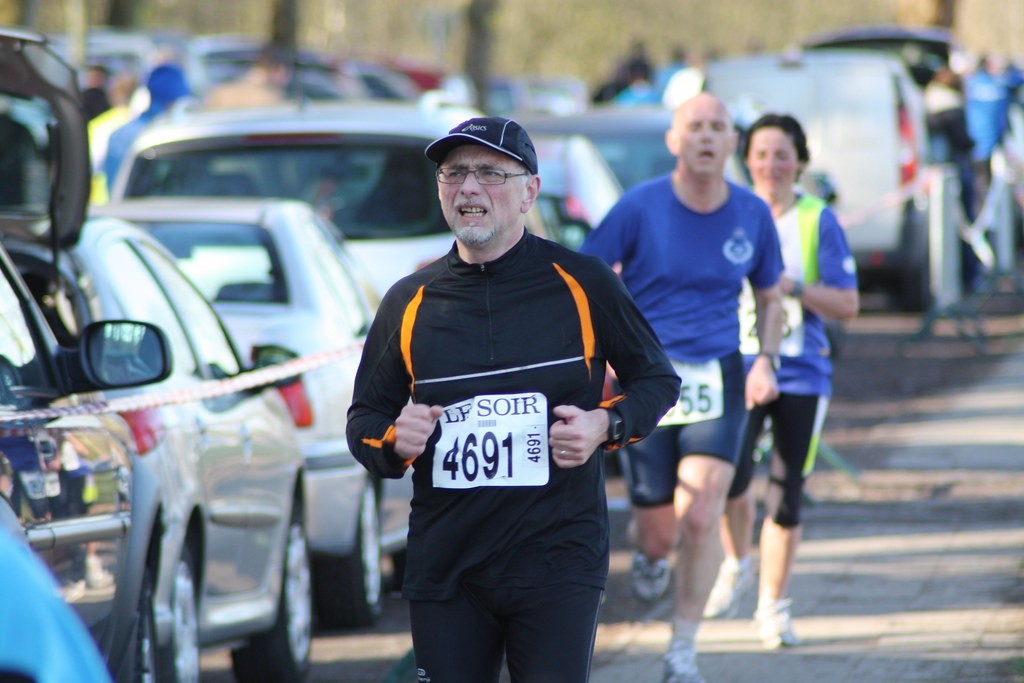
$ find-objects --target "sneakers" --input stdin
[631,545,673,604]
[755,599,797,650]
[662,648,706,683]
[702,556,754,619]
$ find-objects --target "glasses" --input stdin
[435,167,527,185]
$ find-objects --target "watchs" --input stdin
[599,407,623,448]
[757,350,782,372]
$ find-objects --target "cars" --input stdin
[1,24,1024,683]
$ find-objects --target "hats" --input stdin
[425,117,538,174]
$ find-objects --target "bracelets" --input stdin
[792,281,803,296]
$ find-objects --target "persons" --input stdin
[911,51,1024,299]
[84,47,308,204]
[701,112,859,649]
[346,115,682,682]
[573,93,784,683]
